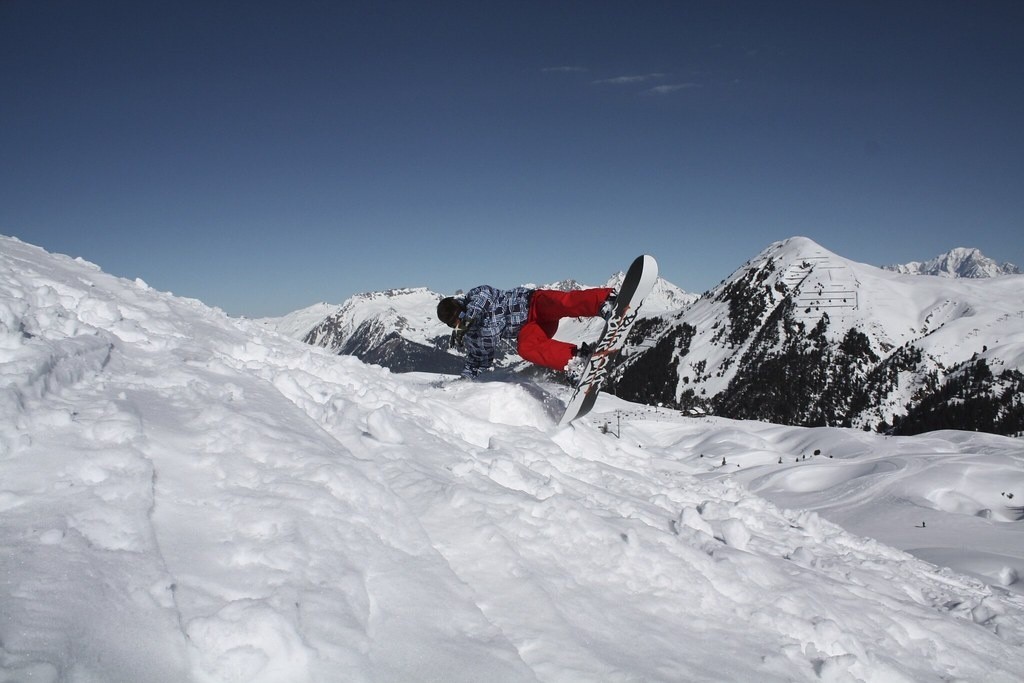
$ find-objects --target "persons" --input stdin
[430,285,619,388]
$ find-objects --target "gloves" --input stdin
[450,324,468,349]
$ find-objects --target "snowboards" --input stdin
[558,254,660,427]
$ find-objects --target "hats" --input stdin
[437,296,458,322]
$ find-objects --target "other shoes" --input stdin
[599,295,617,317]
[567,342,590,384]
